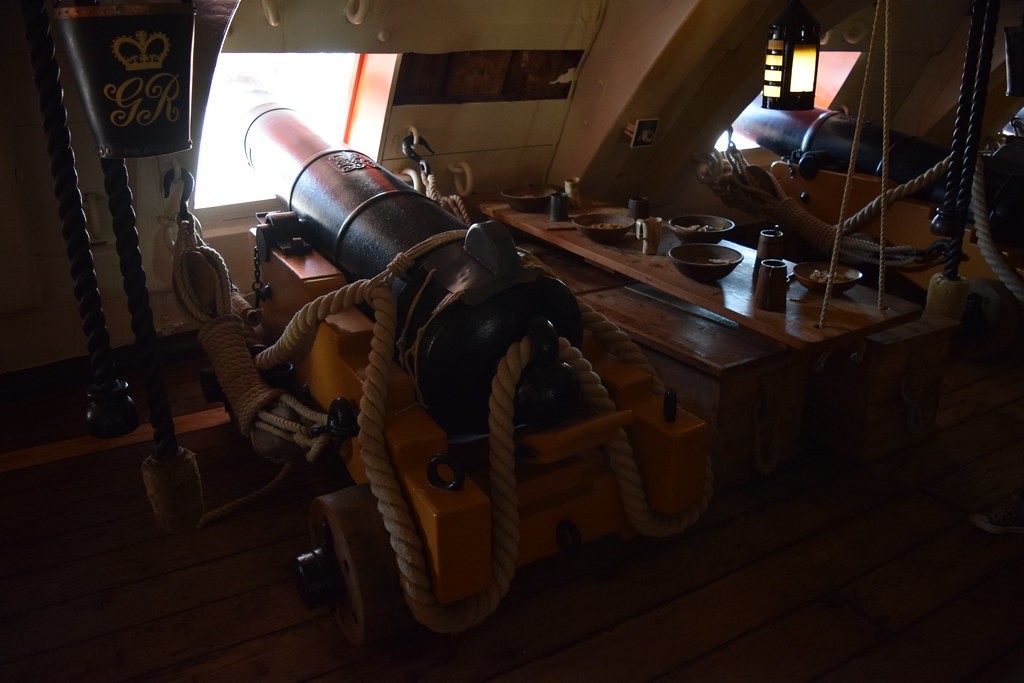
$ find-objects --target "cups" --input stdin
[628,197,649,232]
[564,177,582,209]
[753,230,784,277]
[754,259,787,311]
[549,193,568,221]
[635,217,663,255]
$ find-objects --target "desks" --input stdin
[471,191,924,461]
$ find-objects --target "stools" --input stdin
[804,314,965,469]
[534,245,636,297]
[582,282,810,491]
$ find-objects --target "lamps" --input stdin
[762,0,822,111]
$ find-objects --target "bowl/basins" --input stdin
[572,214,635,243]
[668,244,744,282]
[668,215,735,245]
[792,262,863,294]
[500,186,558,212]
